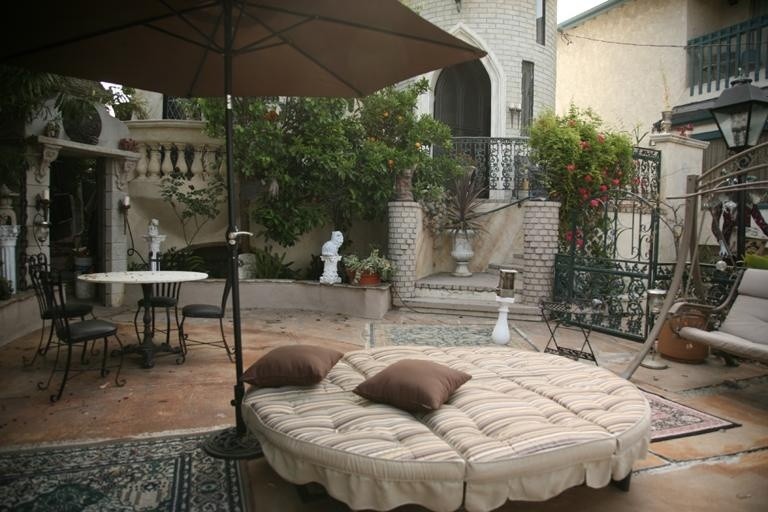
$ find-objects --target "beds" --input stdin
[240,344,653,512]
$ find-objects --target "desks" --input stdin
[538,296,604,366]
[491,295,515,345]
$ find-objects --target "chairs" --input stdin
[22,247,237,403]
[667,265,768,361]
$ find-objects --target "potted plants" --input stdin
[438,167,491,277]
[343,248,391,286]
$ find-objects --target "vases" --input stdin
[655,309,710,364]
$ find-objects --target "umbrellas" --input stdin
[1,1,489,460]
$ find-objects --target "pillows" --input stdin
[240,344,345,384]
[353,356,473,411]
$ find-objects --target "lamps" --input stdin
[118,196,131,235]
[32,188,50,249]
[495,269,518,297]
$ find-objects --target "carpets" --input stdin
[638,383,742,442]
[362,320,540,353]
[0,424,256,512]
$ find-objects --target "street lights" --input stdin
[708,79,768,270]
